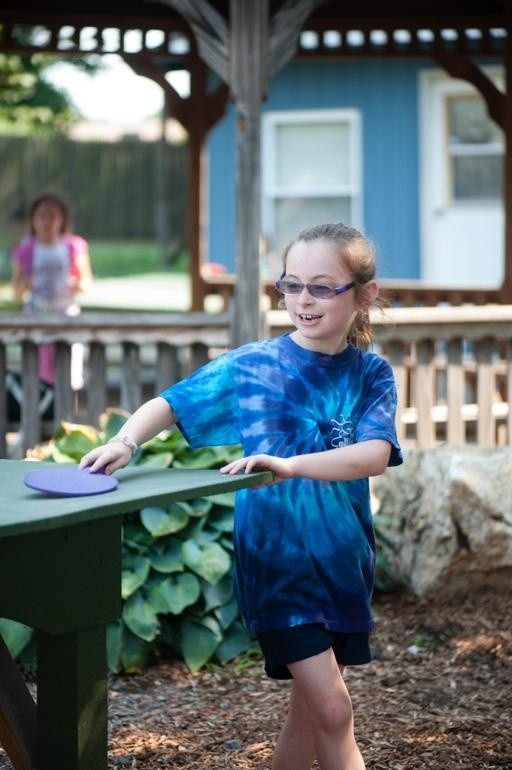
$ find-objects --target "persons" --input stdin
[78,222,404,770]
[12,194,97,417]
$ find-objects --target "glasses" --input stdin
[275,272,354,298]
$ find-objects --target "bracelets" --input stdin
[107,435,137,461]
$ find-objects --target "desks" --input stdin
[0,455,276,770]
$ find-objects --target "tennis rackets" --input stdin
[24,464,118,496]
